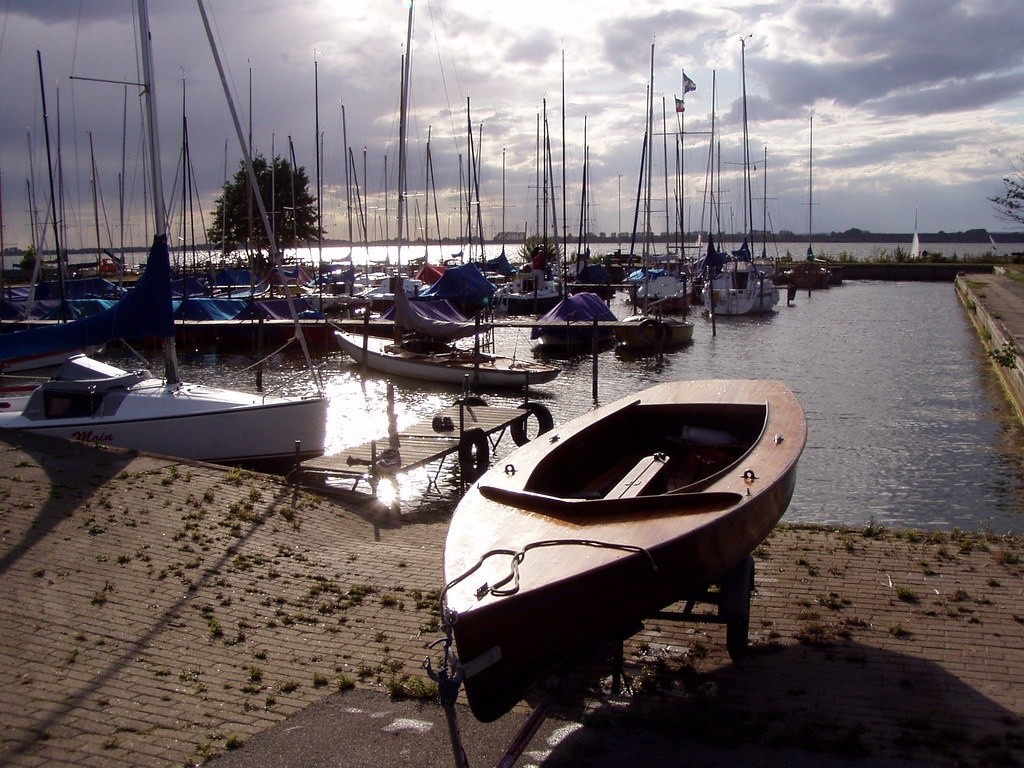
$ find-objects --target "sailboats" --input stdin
[0,0,848,464]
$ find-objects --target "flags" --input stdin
[683,73,696,93]
[675,99,685,112]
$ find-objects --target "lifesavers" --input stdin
[638,320,672,345]
[511,402,554,448]
[453,397,489,407]
[459,428,490,486]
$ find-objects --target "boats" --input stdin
[439,374,809,629]
